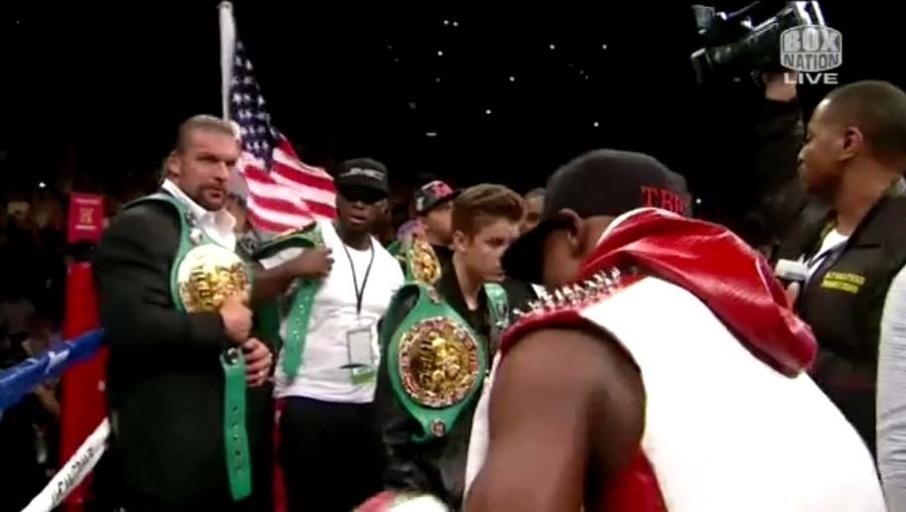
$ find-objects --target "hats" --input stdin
[330,154,390,195]
[496,146,698,282]
[412,176,463,216]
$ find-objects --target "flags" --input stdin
[224,11,340,245]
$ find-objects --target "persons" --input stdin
[2,71,904,512]
